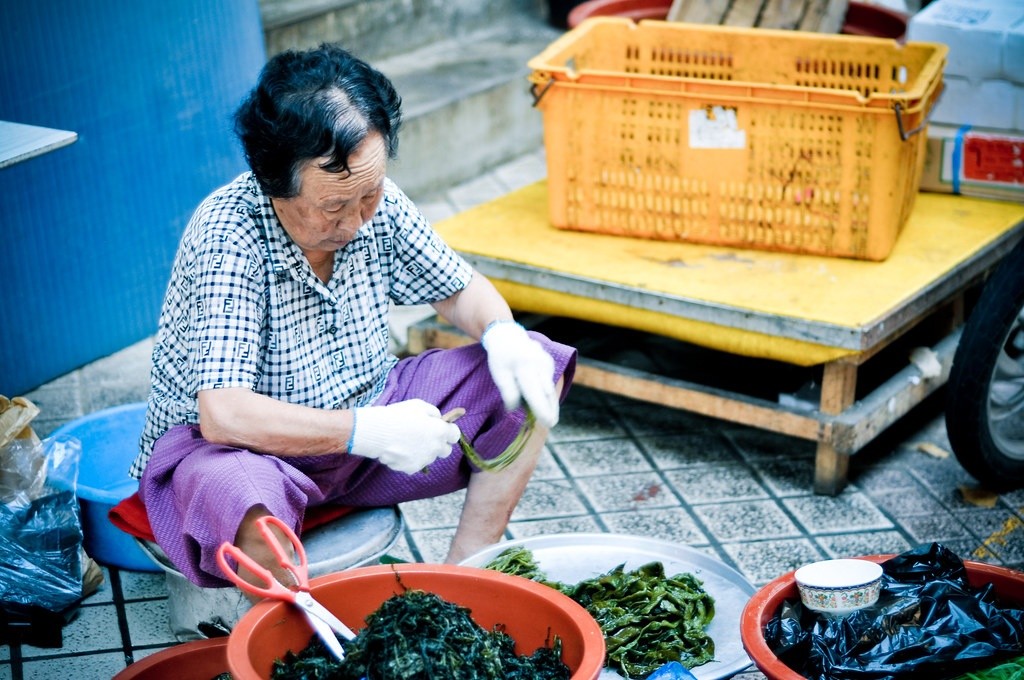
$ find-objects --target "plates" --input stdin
[457,533,758,680]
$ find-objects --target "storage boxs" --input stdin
[529,15,952,262]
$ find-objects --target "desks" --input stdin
[407,178,1024,498]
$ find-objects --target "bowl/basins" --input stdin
[569,0,908,74]
[40,399,605,680]
[741,554,1024,680]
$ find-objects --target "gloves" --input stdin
[348,398,461,474]
[481,319,560,429]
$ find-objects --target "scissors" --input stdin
[215,514,356,664]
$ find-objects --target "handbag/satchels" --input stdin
[0,434,82,613]
[765,542,1024,679]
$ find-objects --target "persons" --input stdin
[130,42,578,608]
